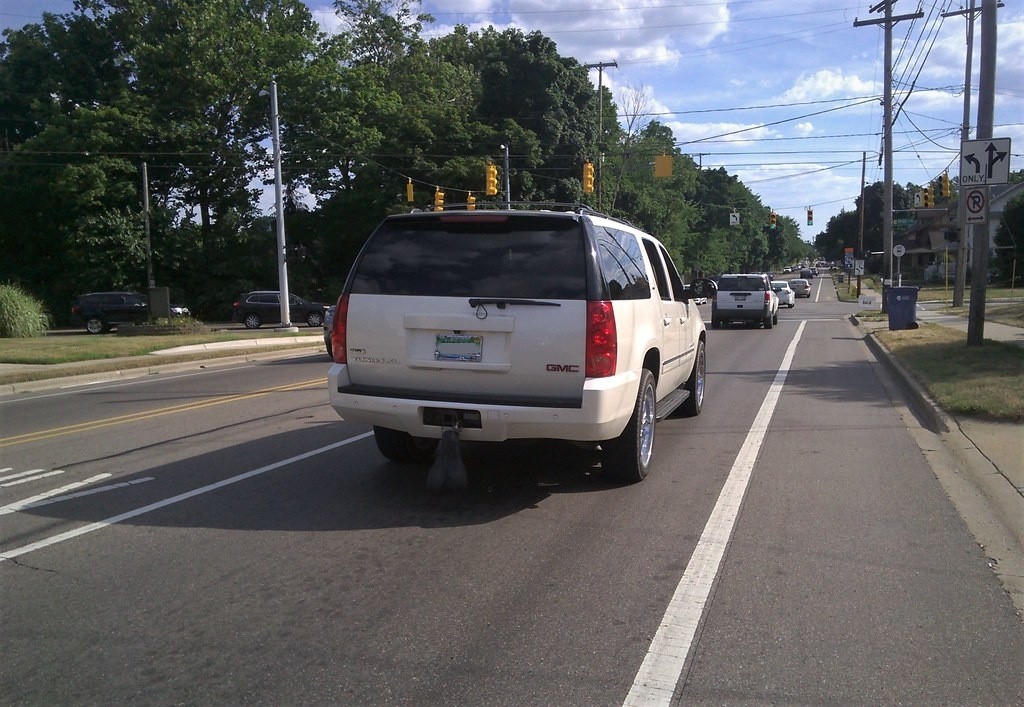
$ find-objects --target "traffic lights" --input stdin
[583,164,594,192]
[467,194,477,211]
[807,209,814,226]
[923,186,934,209]
[770,212,777,231]
[487,164,498,196]
[434,192,444,212]
[939,173,950,197]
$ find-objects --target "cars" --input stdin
[685,284,708,305]
[783,256,838,279]
[789,278,813,297]
[768,280,797,308]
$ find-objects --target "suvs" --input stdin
[711,272,782,329]
[232,290,327,330]
[72,290,193,336]
[327,199,719,485]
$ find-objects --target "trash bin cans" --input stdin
[838,275,844,283]
[886,285,921,330]
